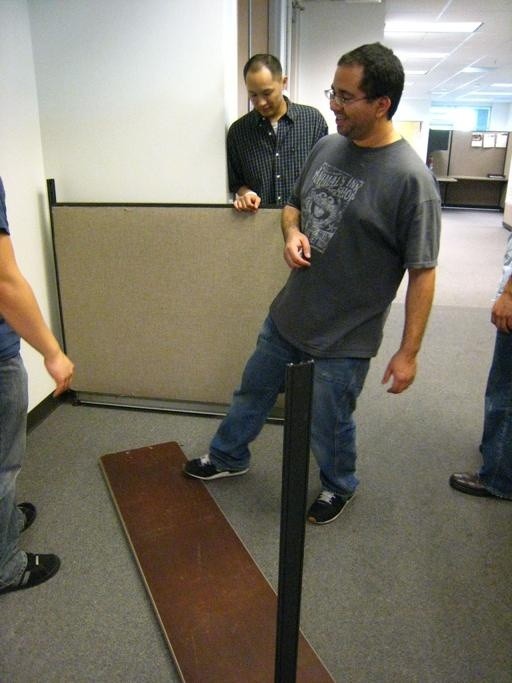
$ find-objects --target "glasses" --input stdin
[324,87,372,110]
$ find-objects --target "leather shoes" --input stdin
[449,469,493,496]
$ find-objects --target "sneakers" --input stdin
[16,501,36,533]
[182,452,250,480]
[1,552,60,595]
[307,488,357,525]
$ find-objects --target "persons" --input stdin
[0,181,76,596]
[184,40,445,525]
[226,52,328,214]
[450,230,512,502]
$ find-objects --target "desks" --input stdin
[433,175,508,212]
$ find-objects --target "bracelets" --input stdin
[244,190,258,197]
